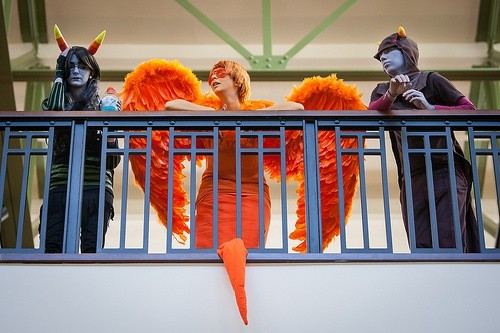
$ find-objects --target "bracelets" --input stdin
[387,90,396,98]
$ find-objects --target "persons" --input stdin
[38,46,122,253]
[368,33,480,253]
[165,60,304,249]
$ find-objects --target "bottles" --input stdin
[98,85,118,143]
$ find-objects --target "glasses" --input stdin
[69,63,89,69]
[209,69,226,85]
